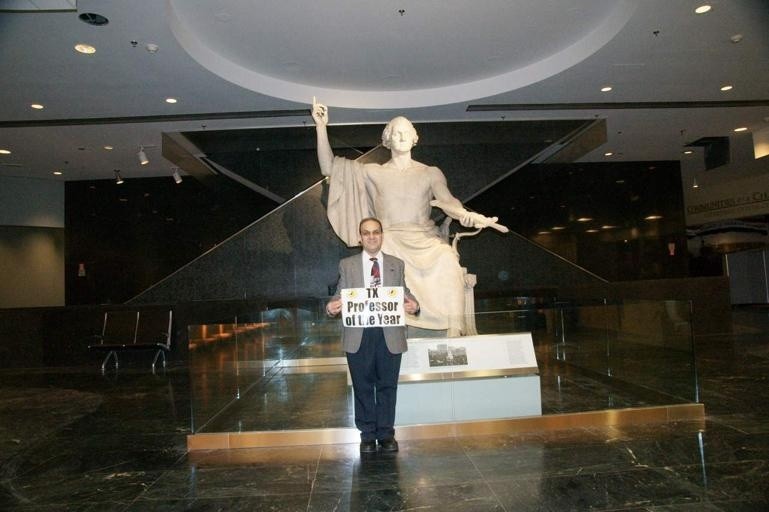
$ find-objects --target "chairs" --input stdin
[85,308,175,375]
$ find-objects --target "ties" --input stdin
[370,258,382,288]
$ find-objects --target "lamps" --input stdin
[133,138,149,167]
[169,166,183,185]
[113,169,124,186]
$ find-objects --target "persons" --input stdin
[310,95,502,339]
[638,250,669,278]
[324,218,421,458]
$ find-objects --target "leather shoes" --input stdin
[360,441,377,453]
[378,437,398,452]
